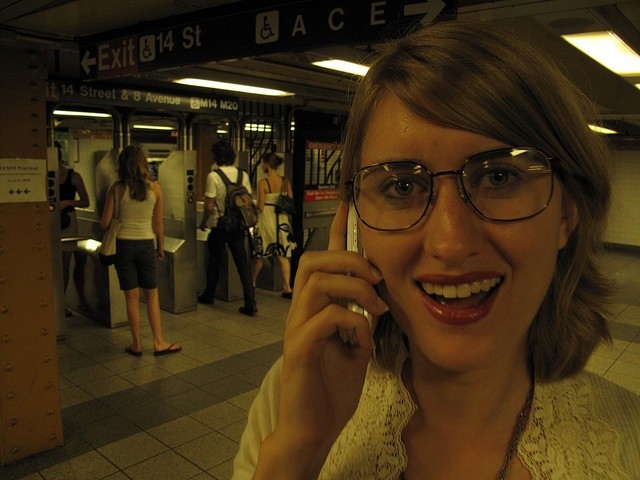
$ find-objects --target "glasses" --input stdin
[345,146,558,232]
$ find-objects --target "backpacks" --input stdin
[212,167,258,232]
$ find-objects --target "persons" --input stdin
[251,153,294,298]
[198,142,258,316]
[55,141,90,316]
[100,145,182,357]
[233,22,639,480]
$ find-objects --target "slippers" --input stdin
[125,345,142,356]
[153,344,182,356]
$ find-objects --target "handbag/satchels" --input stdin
[276,176,296,215]
[98,182,121,256]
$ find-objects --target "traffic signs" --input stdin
[78,1,455,80]
[43,78,240,114]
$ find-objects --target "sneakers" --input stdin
[197,292,214,304]
[239,305,257,316]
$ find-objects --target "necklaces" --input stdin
[398,377,534,480]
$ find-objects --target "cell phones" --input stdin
[339,201,374,346]
